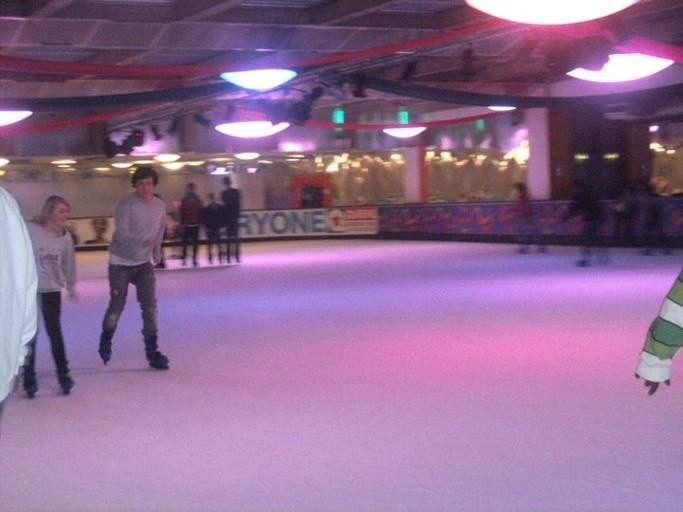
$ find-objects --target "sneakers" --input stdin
[98,343,113,362]
[143,353,170,366]
[27,377,74,393]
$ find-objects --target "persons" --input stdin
[161,213,179,240]
[611,174,638,245]
[84,217,111,244]
[96,165,170,368]
[506,181,547,252]
[150,191,166,268]
[632,265,682,397]
[202,191,226,263]
[633,177,675,256]
[0,182,40,415]
[178,181,202,267]
[558,175,609,265]
[221,176,240,264]
[17,194,80,396]
